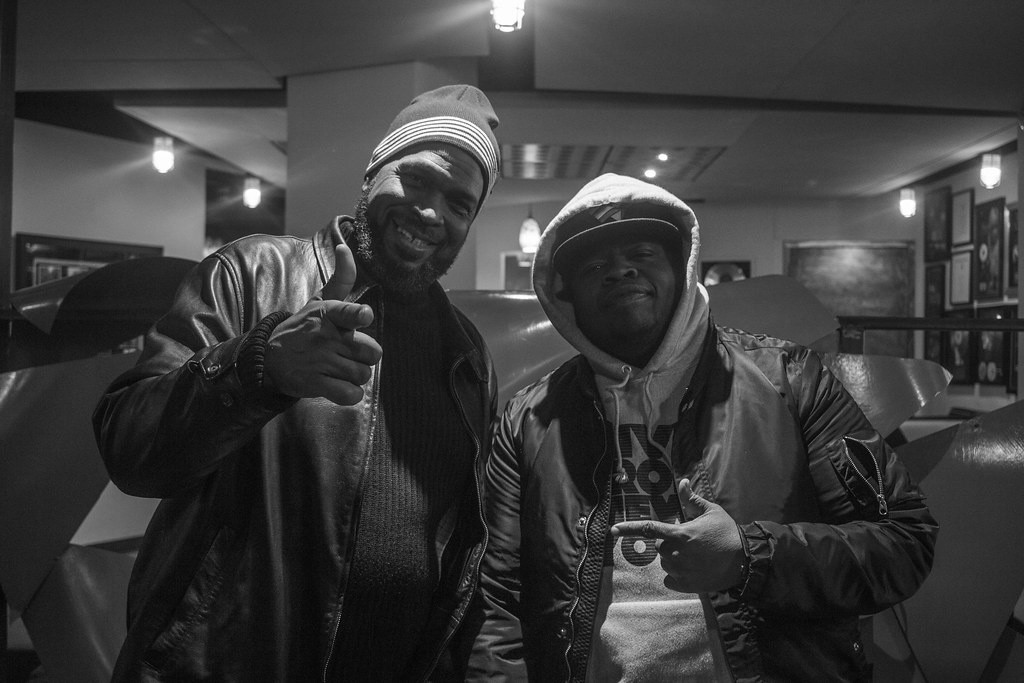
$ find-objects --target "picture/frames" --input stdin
[924,315,945,363]
[924,264,946,316]
[923,185,952,263]
[949,250,973,306]
[942,306,975,386]
[970,197,1005,299]
[951,187,975,248]
[1005,201,1018,298]
[975,305,1016,386]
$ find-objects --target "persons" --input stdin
[454,170,940,682]
[91,84,502,683]
[987,207,999,290]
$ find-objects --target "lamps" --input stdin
[243,177,262,208]
[488,0,526,34]
[519,204,542,253]
[899,186,917,218]
[979,150,1002,189]
[152,136,174,174]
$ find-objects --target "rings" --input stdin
[672,549,680,562]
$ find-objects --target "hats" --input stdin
[551,203,683,270]
[362,85,502,229]
